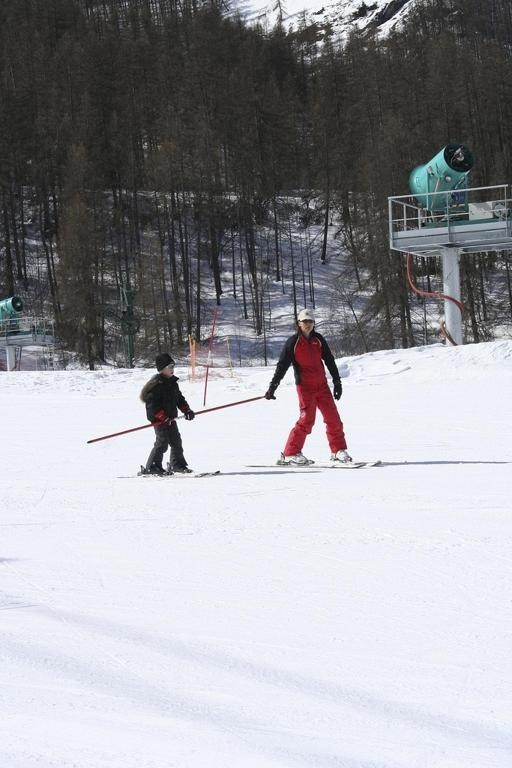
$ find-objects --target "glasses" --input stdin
[165,364,174,369]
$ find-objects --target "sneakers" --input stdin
[145,463,167,475]
[329,449,353,463]
[283,451,307,464]
[168,462,194,473]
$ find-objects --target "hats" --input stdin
[156,351,176,372]
[297,308,316,322]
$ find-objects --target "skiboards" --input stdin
[116,470,222,479]
[246,459,380,468]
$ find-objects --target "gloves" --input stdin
[333,383,343,401]
[265,381,278,400]
[162,415,177,427]
[179,402,195,420]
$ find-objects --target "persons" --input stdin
[141,352,195,473]
[265,308,351,465]
